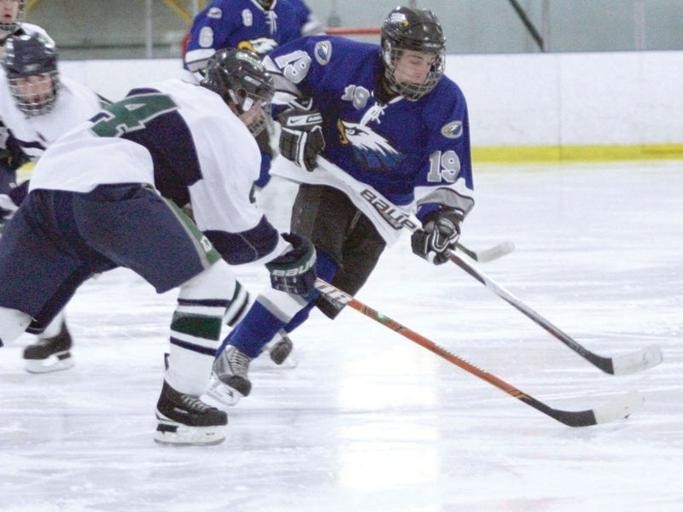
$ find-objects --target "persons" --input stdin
[0,46,319,428]
[182,1,327,209]
[211,5,476,399]
[0,0,48,42]
[0,28,86,359]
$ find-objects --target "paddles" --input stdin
[314,277,644,427]
[316,154,663,374]
[457,229,518,263]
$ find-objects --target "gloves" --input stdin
[409,205,464,268]
[266,232,318,298]
[280,98,327,173]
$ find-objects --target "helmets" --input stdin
[380,7,448,99]
[203,49,272,135]
[3,23,59,113]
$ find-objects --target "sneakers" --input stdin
[157,378,228,427]
[214,335,292,397]
[22,322,72,358]
